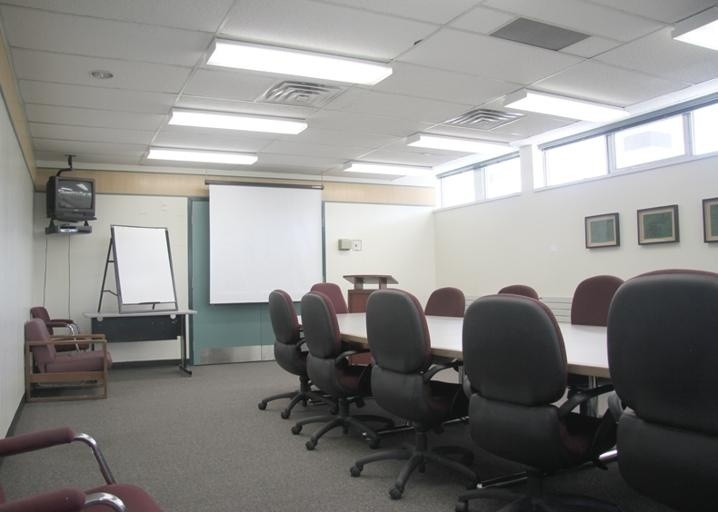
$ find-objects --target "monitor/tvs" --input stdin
[46,176,97,222]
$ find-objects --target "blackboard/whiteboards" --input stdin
[111,224,177,305]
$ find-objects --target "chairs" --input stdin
[291,291,395,449]
[424,288,467,319]
[455,293,626,511]
[570,274,625,326]
[498,284,539,302]
[29,307,90,353]
[258,289,341,419]
[0,427,164,511]
[607,266,717,512]
[23,319,113,402]
[350,288,481,500]
[309,283,349,315]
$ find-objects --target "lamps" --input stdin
[342,160,434,178]
[503,88,631,126]
[144,145,261,166]
[405,131,516,157]
[203,37,393,87]
[167,106,310,136]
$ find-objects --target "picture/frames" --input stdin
[583,212,620,249]
[636,203,680,246]
[702,197,718,243]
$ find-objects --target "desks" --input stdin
[296,310,611,424]
[81,308,197,376]
